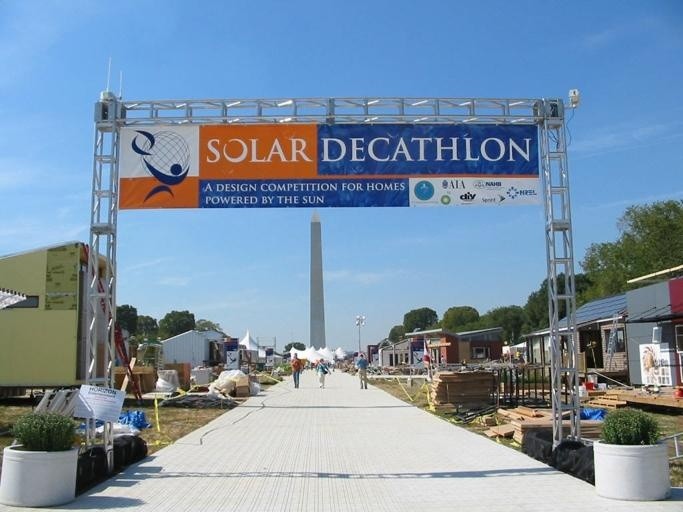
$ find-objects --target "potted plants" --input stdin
[592,408,671,500]
[1,410,82,507]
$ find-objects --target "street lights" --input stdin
[354,312,366,357]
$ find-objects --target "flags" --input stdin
[422,339,430,365]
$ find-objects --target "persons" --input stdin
[315,358,330,388]
[356,354,368,389]
[290,353,301,388]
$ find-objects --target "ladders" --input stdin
[81,243,145,407]
